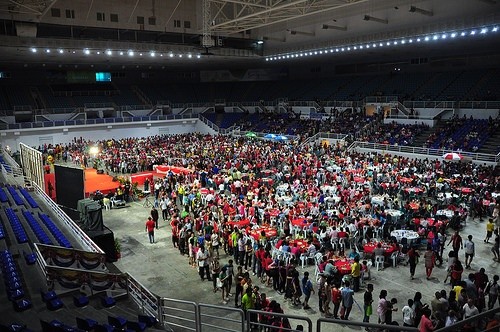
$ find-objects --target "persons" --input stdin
[17,109,500,331]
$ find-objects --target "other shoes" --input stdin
[171,240,242,291]
[225,296,229,301]
[223,301,227,305]
[243,239,500,320]
[228,293,233,296]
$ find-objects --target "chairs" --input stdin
[0,146,162,332]
[0,77,499,165]
